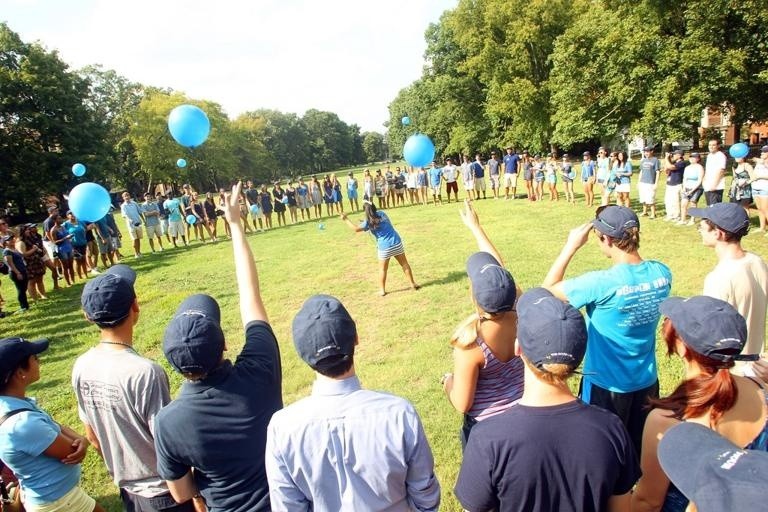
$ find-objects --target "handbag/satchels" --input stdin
[395,175,402,189]
[375,182,382,196]
[587,161,594,184]
[0,409,43,512]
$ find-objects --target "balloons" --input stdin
[403,133,435,168]
[186,215,196,224]
[68,182,111,222]
[250,205,257,212]
[402,116,410,124]
[72,164,85,177]
[168,104,211,149]
[319,224,323,228]
[730,143,750,159]
[282,196,288,204]
[177,159,186,167]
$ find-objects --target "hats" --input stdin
[82,264,136,326]
[535,154,540,159]
[690,152,699,158]
[4,234,15,241]
[156,192,161,196]
[311,174,316,177]
[467,252,517,314]
[670,150,683,155]
[491,151,495,152]
[274,181,280,185]
[48,206,58,212]
[507,146,512,149]
[642,146,654,151]
[0,336,49,375]
[687,202,749,232]
[377,169,380,172]
[25,223,38,228]
[657,422,767,512]
[661,294,760,365]
[522,150,527,154]
[167,191,170,193]
[183,184,190,188]
[583,151,590,155]
[590,203,640,238]
[67,210,71,214]
[144,192,149,196]
[293,295,354,373]
[163,295,225,381]
[365,168,368,171]
[548,153,553,157]
[562,154,569,158]
[446,158,450,160]
[760,146,768,152]
[516,288,599,377]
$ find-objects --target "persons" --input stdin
[703,139,727,206]
[614,151,633,207]
[50,214,76,287]
[428,161,443,207]
[751,146,768,233]
[330,174,345,216]
[580,151,596,208]
[295,176,313,222]
[239,189,255,235]
[142,193,166,254]
[544,153,558,202]
[441,198,524,459]
[340,201,420,296]
[460,155,475,202]
[452,287,642,512]
[84,205,124,274]
[558,154,575,204]
[308,175,323,219]
[71,265,195,512]
[42,206,62,280]
[0,262,9,302]
[685,203,768,377]
[0,219,16,248]
[597,147,608,206]
[61,210,88,280]
[487,152,501,198]
[726,158,753,217]
[604,152,617,206]
[676,151,703,226]
[664,149,686,222]
[180,184,198,245]
[0,338,107,512]
[503,146,521,200]
[203,192,218,239]
[259,184,273,229]
[530,155,546,201]
[191,192,216,243]
[246,181,264,234]
[272,181,286,227]
[522,150,536,201]
[0,235,30,310]
[473,154,486,200]
[637,146,660,219]
[15,225,50,301]
[156,181,283,512]
[120,192,146,259]
[266,295,441,511]
[322,174,334,216]
[163,191,188,248]
[218,188,232,241]
[156,191,178,244]
[416,168,429,206]
[630,296,768,512]
[442,158,460,203]
[24,223,63,291]
[540,204,671,465]
[346,171,359,213]
[285,180,298,224]
[363,166,419,209]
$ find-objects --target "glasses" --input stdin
[595,204,618,219]
